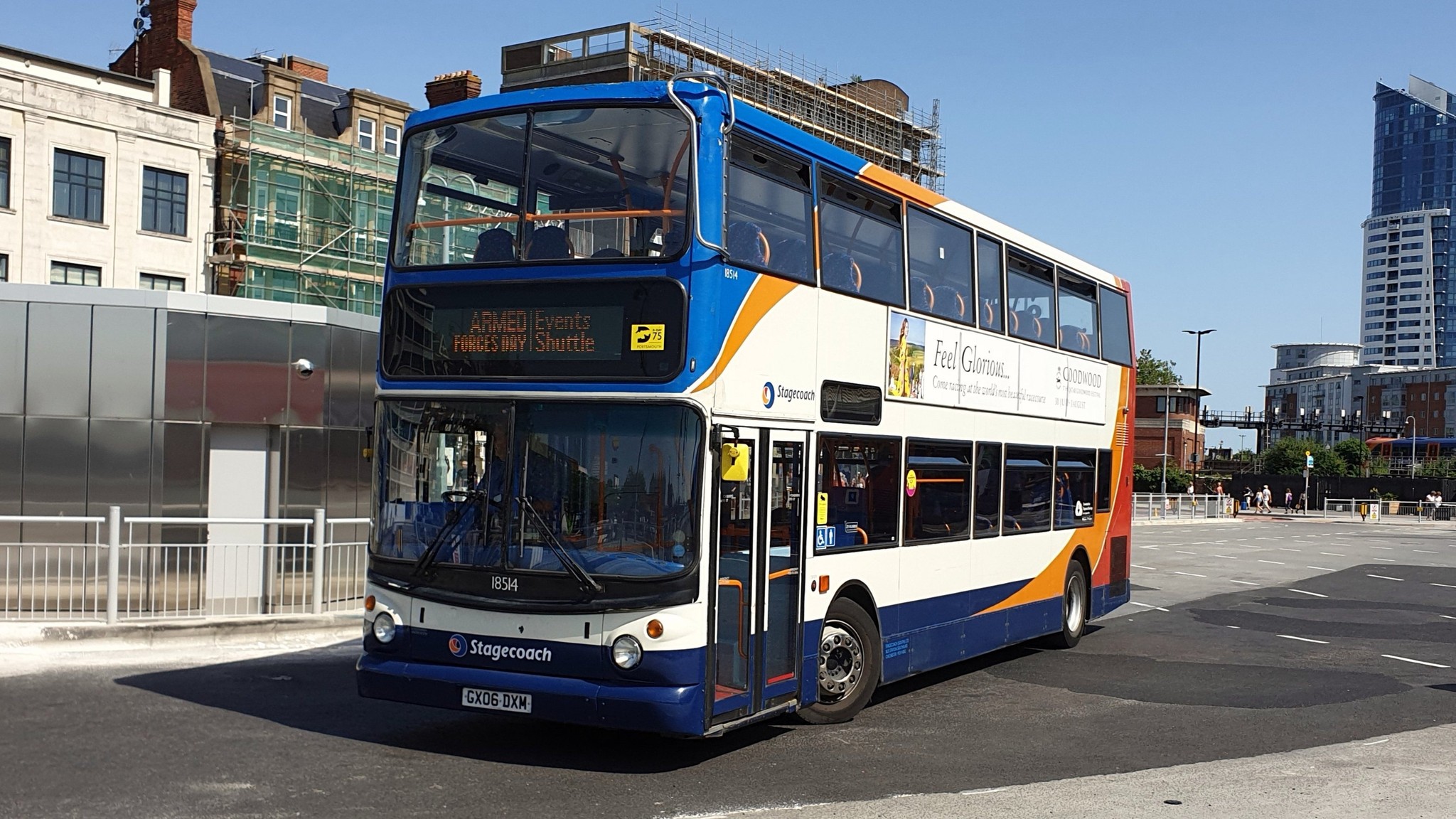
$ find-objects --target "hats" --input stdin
[1263,485,1268,489]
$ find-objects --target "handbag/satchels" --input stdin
[1295,504,1300,509]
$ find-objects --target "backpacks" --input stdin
[1288,494,1292,501]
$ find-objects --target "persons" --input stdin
[888,318,924,400]
[722,467,793,513]
[1252,485,1272,514]
[1296,490,1309,515]
[1284,488,1294,514]
[1187,481,1199,505]
[475,414,562,546]
[1243,485,1254,510]
[1426,491,1443,520]
[833,470,870,488]
[1214,482,1224,501]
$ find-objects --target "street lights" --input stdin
[1181,328,1216,495]
[1403,415,1416,478]
[1353,395,1363,477]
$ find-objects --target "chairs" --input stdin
[833,464,1073,547]
[472,220,1092,356]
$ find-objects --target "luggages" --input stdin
[1241,496,1246,510]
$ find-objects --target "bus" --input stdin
[351,67,1138,743]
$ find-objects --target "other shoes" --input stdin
[1296,510,1298,513]
[1268,511,1272,513]
[1291,510,1293,513]
[1285,512,1287,514]
